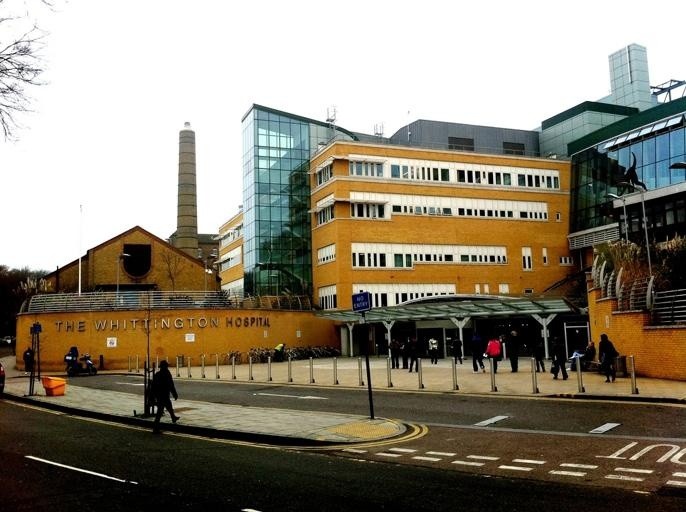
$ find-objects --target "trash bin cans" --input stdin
[41,376,66,395]
[615,357,627,377]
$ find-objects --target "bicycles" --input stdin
[225,344,341,364]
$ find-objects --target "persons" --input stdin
[274,343,286,362]
[570,341,596,371]
[389,330,569,380]
[22,347,35,376]
[151,359,181,434]
[598,333,619,382]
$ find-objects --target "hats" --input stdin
[158,360,172,368]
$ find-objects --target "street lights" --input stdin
[116,254,130,310]
[608,182,652,276]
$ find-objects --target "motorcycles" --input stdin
[64,352,97,377]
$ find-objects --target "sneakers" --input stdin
[153,416,181,433]
